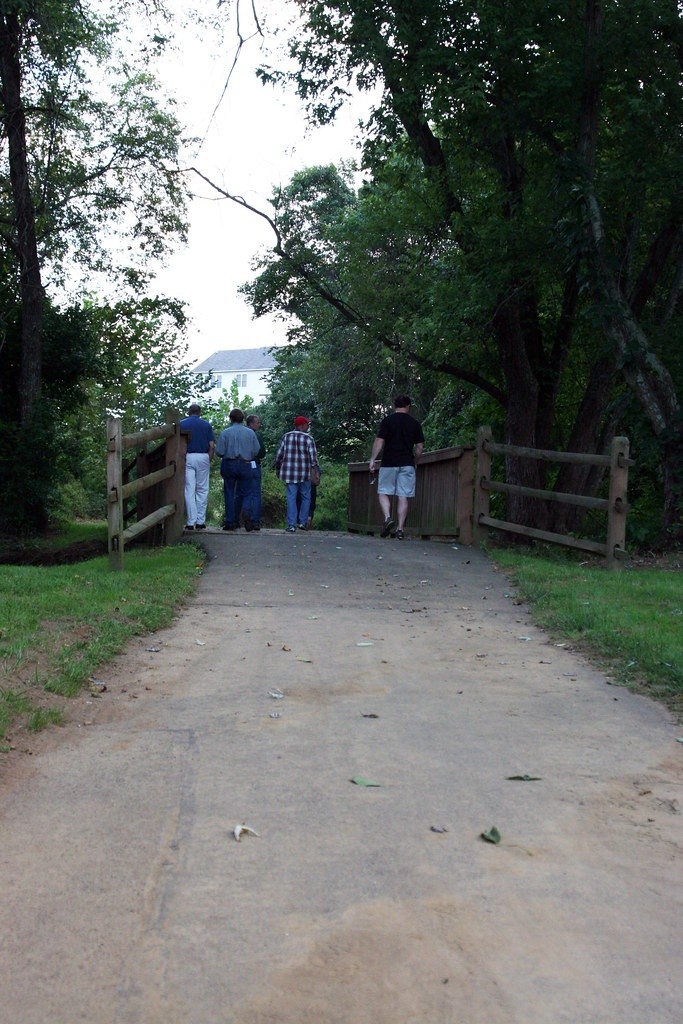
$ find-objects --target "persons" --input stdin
[214,409,266,532]
[180,405,214,531]
[273,416,320,532]
[368,396,425,539]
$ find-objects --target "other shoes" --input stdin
[196,524,206,529]
[391,530,404,540]
[299,523,308,530]
[185,526,193,530]
[308,520,314,529]
[220,519,260,532]
[380,517,394,538]
[285,526,295,532]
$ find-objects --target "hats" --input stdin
[294,416,311,426]
